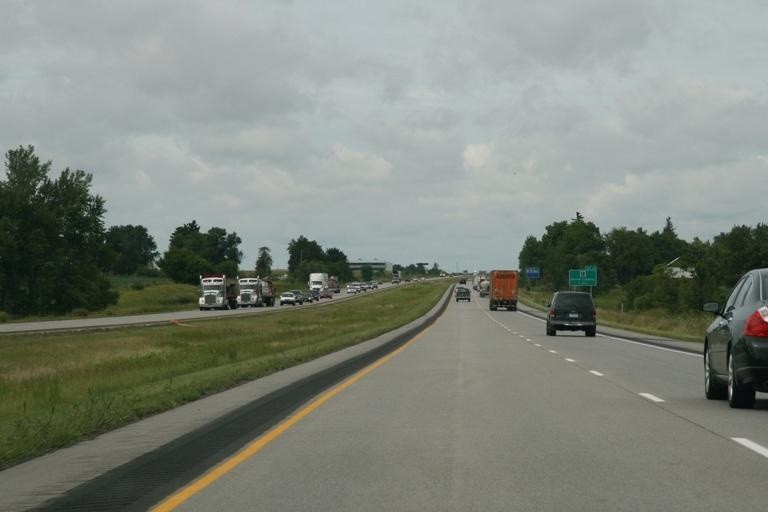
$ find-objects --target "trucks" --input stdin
[236,274,275,307]
[489,269,520,313]
[307,272,329,291]
[198,273,238,311]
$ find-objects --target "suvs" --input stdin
[545,289,597,339]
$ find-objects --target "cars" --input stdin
[279,289,333,306]
[456,287,472,302]
[699,267,767,409]
